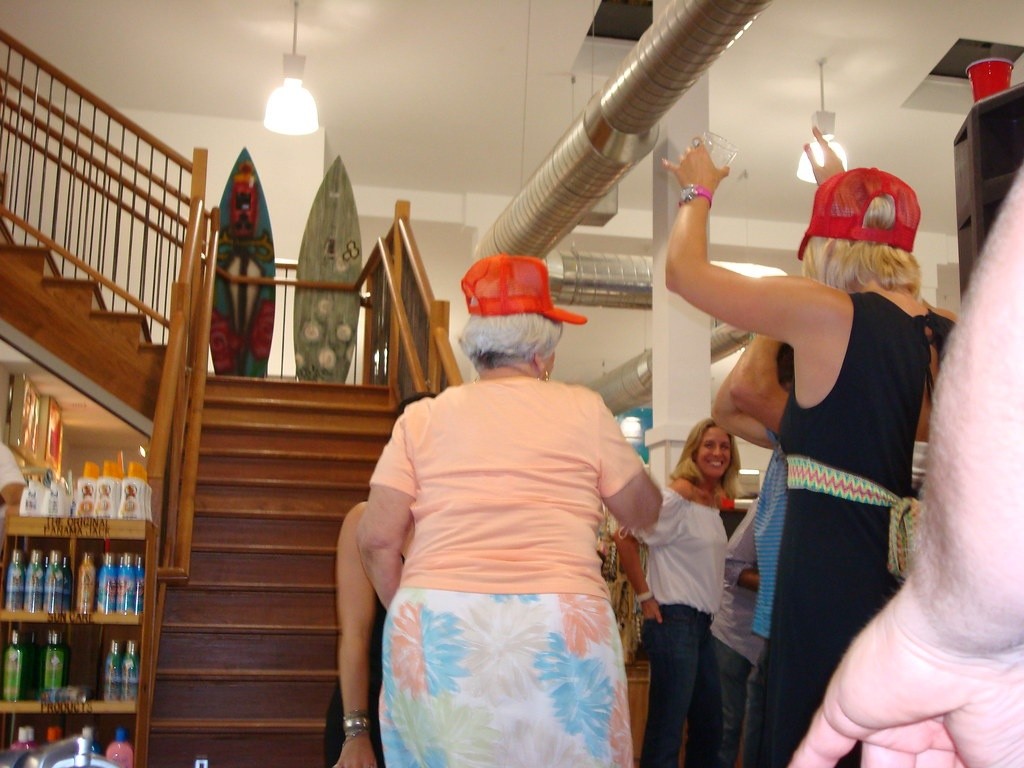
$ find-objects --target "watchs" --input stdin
[678,185,714,209]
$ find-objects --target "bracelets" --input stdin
[636,589,655,603]
[342,710,370,738]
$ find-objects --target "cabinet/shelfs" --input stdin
[0,513,158,768]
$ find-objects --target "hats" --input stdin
[798,167,920,261]
[461,253,588,325]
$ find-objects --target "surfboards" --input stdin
[292,154,363,385]
[210,145,276,378]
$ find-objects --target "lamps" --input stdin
[796,59,847,185]
[263,1,319,137]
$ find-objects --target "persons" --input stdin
[353,254,665,768]
[613,419,741,768]
[661,126,1024,768]
[324,392,463,768]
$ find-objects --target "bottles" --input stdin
[104,640,140,703]
[11,726,135,768]
[4,547,145,615]
[3,626,68,703]
[21,461,153,522]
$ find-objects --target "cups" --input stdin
[700,132,737,169]
[966,57,1014,104]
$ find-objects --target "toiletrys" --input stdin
[5,548,145,616]
[9,725,135,768]
[3,628,70,701]
[102,640,139,704]
[20,457,153,524]
